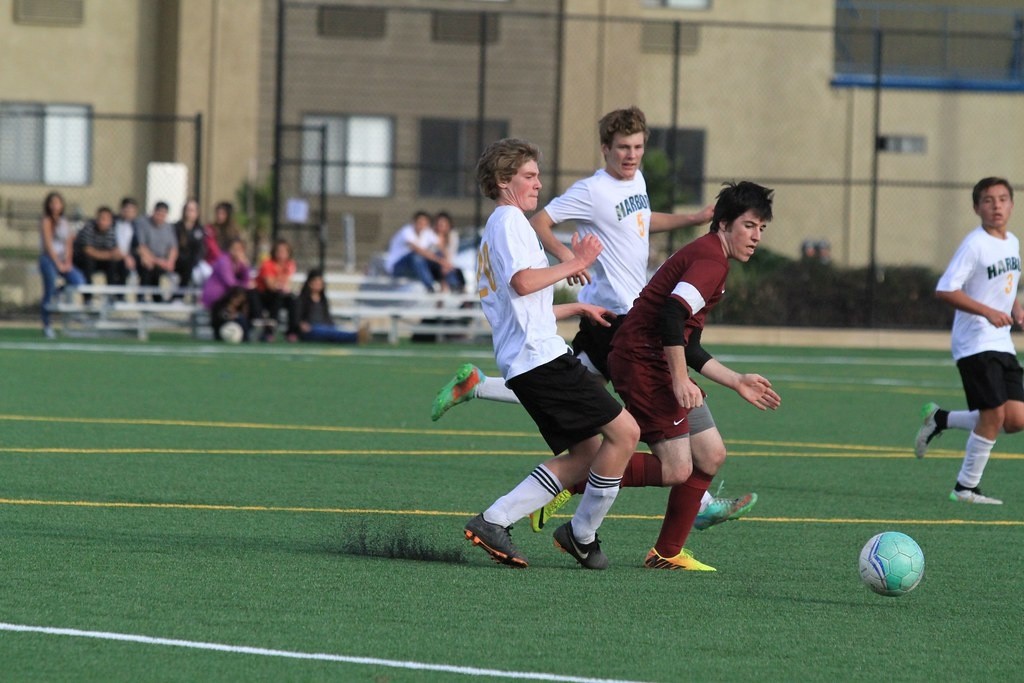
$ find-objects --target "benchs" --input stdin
[42,265,494,344]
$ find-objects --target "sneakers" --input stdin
[645,546,717,572]
[553,521,609,570]
[431,363,483,422]
[950,480,1004,505]
[913,401,949,460]
[465,512,529,569]
[694,480,758,531]
[529,489,572,532]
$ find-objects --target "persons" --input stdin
[203,238,373,346]
[463,138,641,569]
[384,210,465,293]
[430,106,759,531]
[532,181,781,575]
[74,198,243,304]
[914,176,1024,505]
[39,192,85,339]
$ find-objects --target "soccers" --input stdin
[219,321,244,347]
[859,531,925,597]
